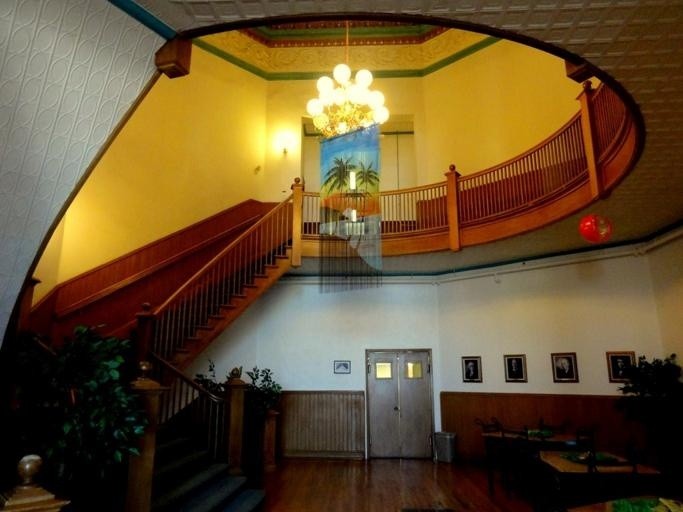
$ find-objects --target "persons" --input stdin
[508,358,523,379]
[613,359,629,379]
[466,362,478,379]
[556,357,574,379]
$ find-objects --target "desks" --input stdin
[478,417,682,510]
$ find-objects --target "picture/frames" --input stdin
[502,352,528,384]
[550,350,580,384]
[333,359,351,374]
[461,354,483,384]
[605,349,639,385]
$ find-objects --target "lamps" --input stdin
[304,20,389,139]
